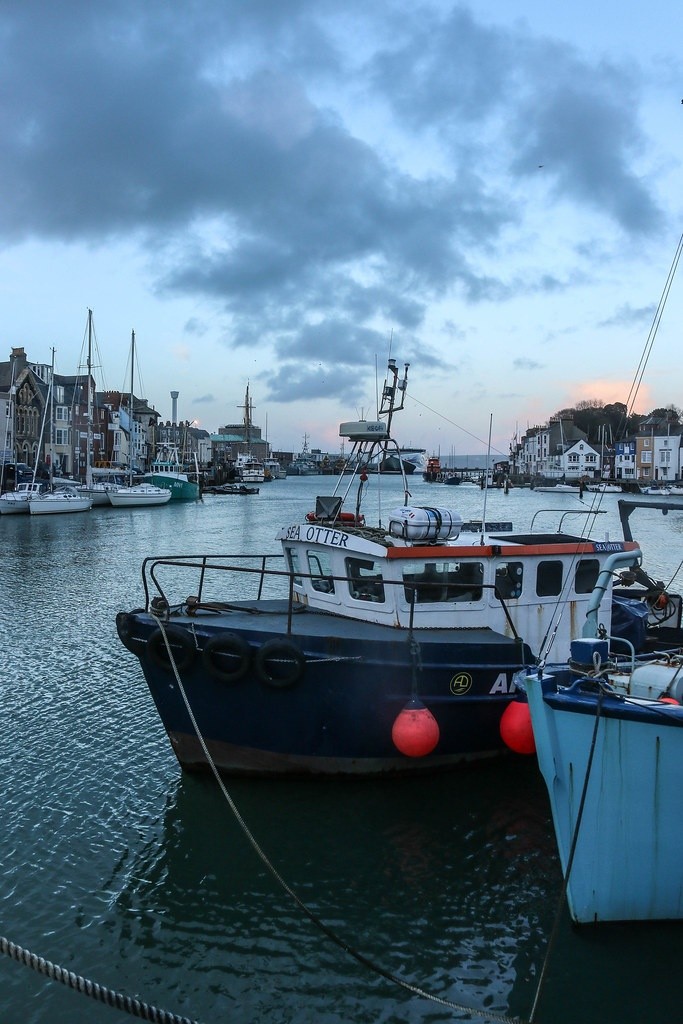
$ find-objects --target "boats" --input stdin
[116,324,683,780]
[637,483,683,496]
[585,482,623,493]
[524,544,683,930]
[0,303,321,517]
[532,484,581,494]
[443,477,461,485]
[380,454,416,475]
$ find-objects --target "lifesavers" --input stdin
[129,608,146,615]
[252,638,306,689]
[306,512,365,529]
[202,632,253,684]
[116,612,145,657]
[145,625,198,677]
